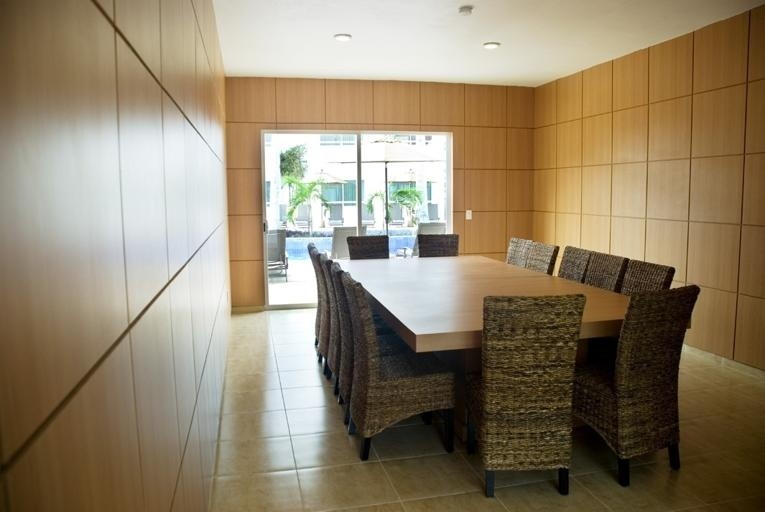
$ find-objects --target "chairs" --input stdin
[266,228,289,284]
